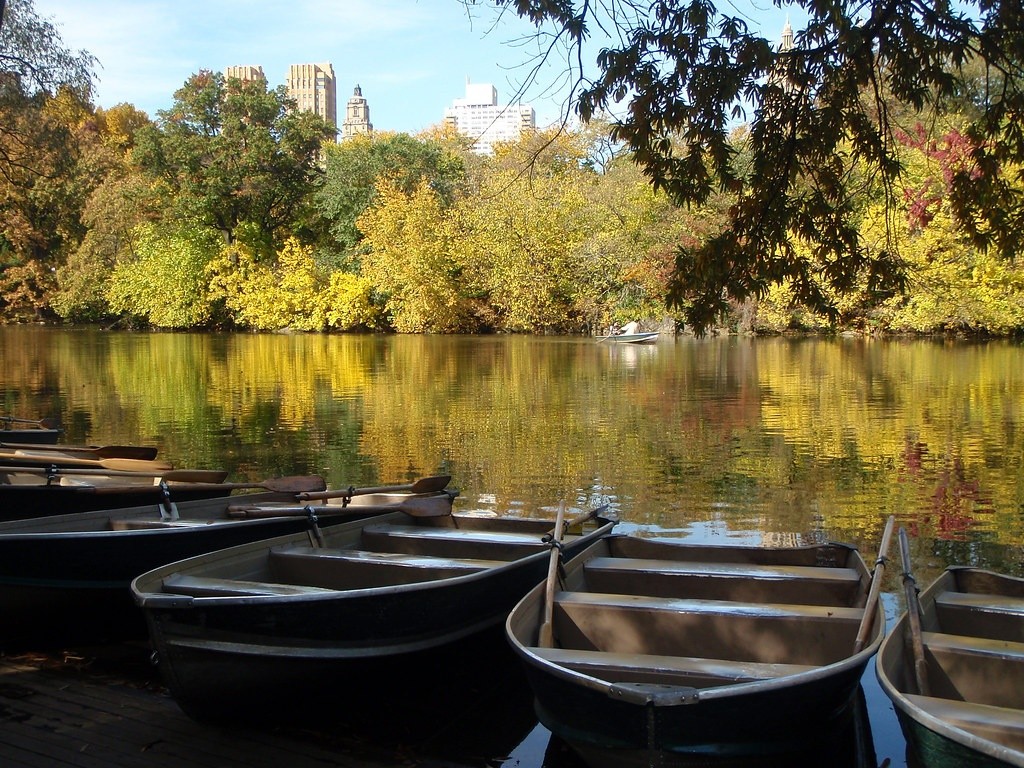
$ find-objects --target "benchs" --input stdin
[526,646,820,690]
[586,558,858,608]
[269,544,514,591]
[109,515,228,532]
[165,575,338,598]
[904,589,1024,751]
[547,591,866,666]
[364,524,548,562]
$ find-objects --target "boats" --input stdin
[875,561,1024,768]
[0,427,462,636]
[595,320,660,344]
[128,490,618,733]
[505,529,888,768]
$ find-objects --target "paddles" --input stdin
[227,492,452,520]
[1,465,230,485]
[601,326,625,342]
[0,452,174,474]
[540,502,611,544]
[0,442,158,462]
[849,512,896,658]
[293,475,452,502]
[76,474,328,495]
[536,498,566,648]
[0,416,57,429]
[896,525,933,696]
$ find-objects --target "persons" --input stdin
[620,317,640,335]
